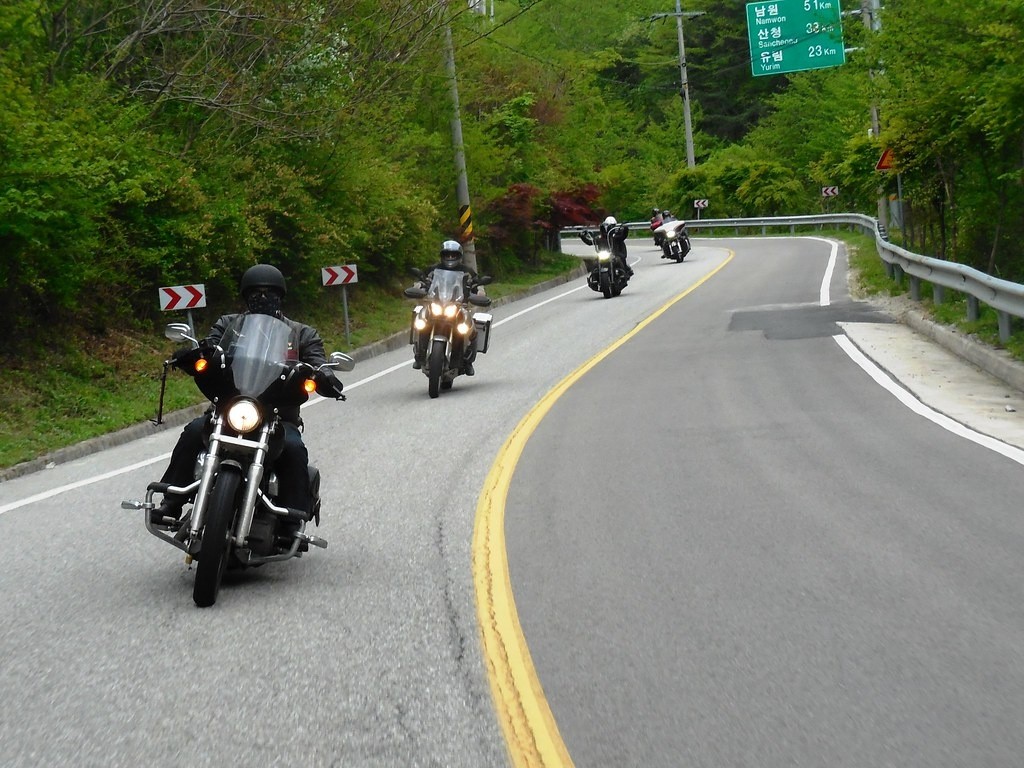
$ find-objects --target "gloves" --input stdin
[174,349,189,363]
[324,376,344,396]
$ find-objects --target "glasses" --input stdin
[248,287,282,304]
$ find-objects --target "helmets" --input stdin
[240,264,287,300]
[441,240,462,269]
[652,208,661,215]
[605,215,616,226]
[663,210,670,218]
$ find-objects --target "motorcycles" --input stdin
[404,267,492,399]
[120,310,355,608]
[577,223,634,298]
[651,213,690,263]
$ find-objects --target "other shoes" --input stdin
[282,522,308,551]
[413,360,421,369]
[152,499,181,525]
[465,362,475,376]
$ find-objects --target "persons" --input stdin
[414,241,487,376]
[151,264,344,552]
[650,208,688,257]
[578,216,632,288]
